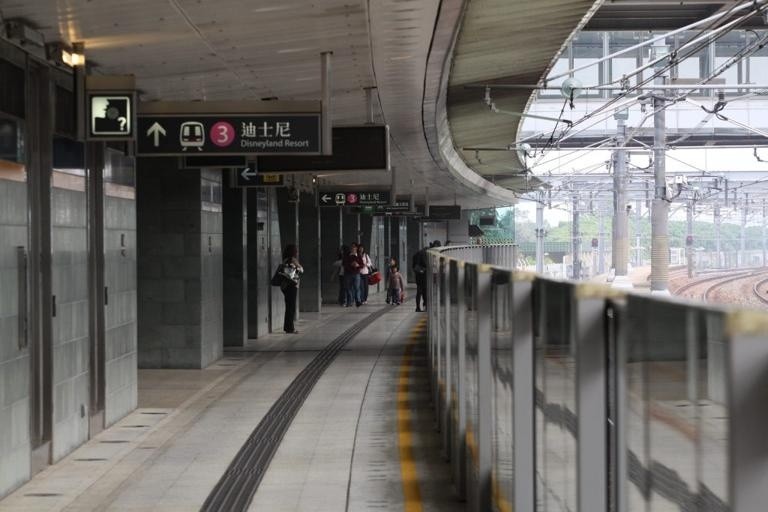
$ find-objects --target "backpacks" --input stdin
[271,257,298,287]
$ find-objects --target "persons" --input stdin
[430,240,441,285]
[271,243,305,334]
[476,237,482,244]
[444,240,452,246]
[413,246,427,312]
[358,244,372,305]
[386,259,397,305]
[341,242,363,308]
[333,245,350,308]
[385,265,405,305]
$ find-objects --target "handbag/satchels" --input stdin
[368,270,382,285]
